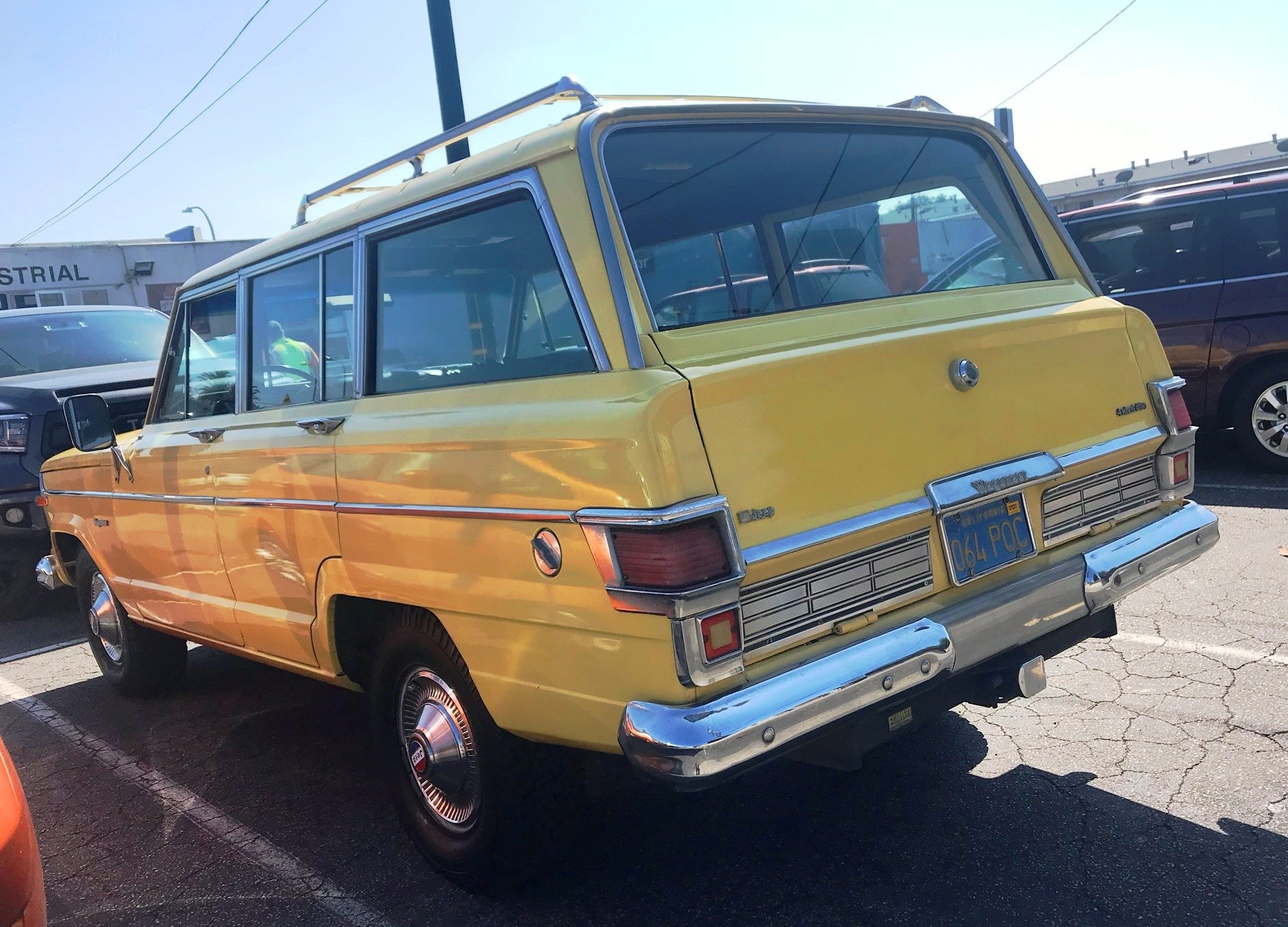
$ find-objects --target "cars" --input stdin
[35,75,1240,860]
[0,305,244,623]
[917,167,1288,475]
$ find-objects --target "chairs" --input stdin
[1124,230,1179,292]
[1077,242,1119,296]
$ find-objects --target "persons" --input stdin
[267,319,320,378]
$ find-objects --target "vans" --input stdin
[653,258,899,332]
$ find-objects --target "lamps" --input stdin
[134,261,154,276]
